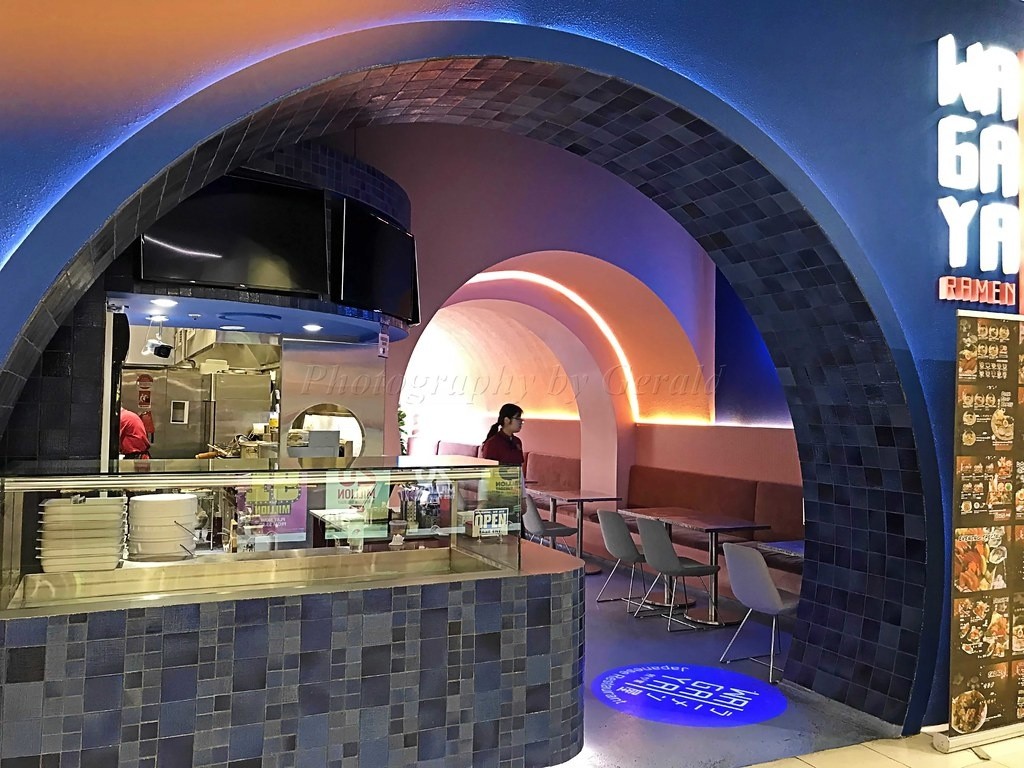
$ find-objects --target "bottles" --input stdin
[439,496,450,527]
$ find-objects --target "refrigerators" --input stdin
[201,372,271,459]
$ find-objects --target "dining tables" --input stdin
[526,484,623,575]
[617,505,773,627]
[757,538,804,558]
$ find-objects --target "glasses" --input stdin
[508,417,525,424]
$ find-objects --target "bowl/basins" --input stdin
[35,497,128,573]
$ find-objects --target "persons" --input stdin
[119,406,151,459]
[477,403,528,538]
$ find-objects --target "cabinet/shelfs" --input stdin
[0,454,524,617]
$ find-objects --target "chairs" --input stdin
[595,508,657,614]
[521,493,578,557]
[718,543,799,684]
[633,517,727,635]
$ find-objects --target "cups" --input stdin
[389,519,408,542]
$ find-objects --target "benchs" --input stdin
[436,441,479,457]
[736,478,806,543]
[619,464,758,556]
[525,453,582,517]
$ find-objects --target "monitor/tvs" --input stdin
[140,166,423,327]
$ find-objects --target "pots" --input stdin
[257,442,279,459]
[238,440,268,459]
